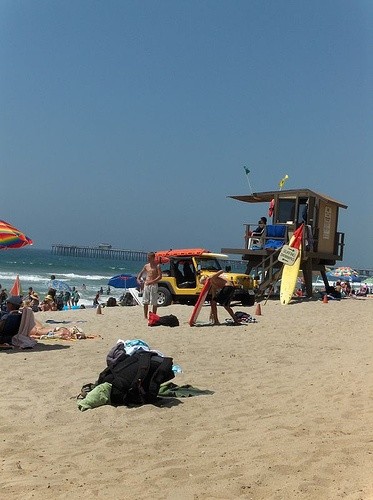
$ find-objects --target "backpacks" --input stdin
[80,347,175,407]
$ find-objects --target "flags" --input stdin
[9,278,19,296]
[279,175,289,188]
[243,166,250,175]
[291,224,303,250]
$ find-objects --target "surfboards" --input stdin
[42,279,75,293]
[280,225,303,305]
[201,253,229,259]
[190,278,211,326]
[129,288,144,305]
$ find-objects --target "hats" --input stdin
[30,293,40,300]
[45,294,53,301]
[6,296,23,305]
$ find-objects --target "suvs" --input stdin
[156,249,258,308]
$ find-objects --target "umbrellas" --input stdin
[329,265,373,283]
[108,274,138,295]
[0,220,33,250]
[273,265,331,278]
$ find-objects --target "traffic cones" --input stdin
[254,303,262,316]
[323,294,328,303]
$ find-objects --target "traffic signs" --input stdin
[278,245,299,266]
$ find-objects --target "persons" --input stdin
[333,277,373,296]
[137,252,162,318]
[22,275,111,311]
[0,288,8,304]
[200,270,238,326]
[0,296,23,345]
[302,199,309,222]
[252,216,267,244]
